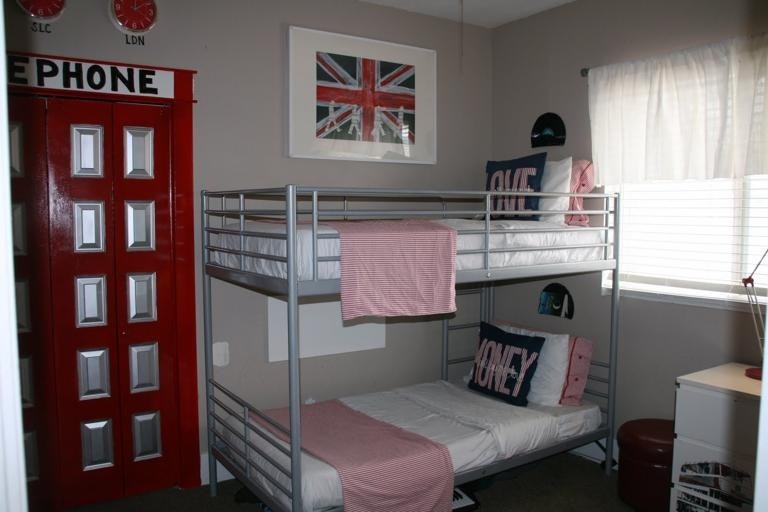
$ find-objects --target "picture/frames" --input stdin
[289,27,438,163]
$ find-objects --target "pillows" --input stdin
[462,321,592,408]
[481,151,596,226]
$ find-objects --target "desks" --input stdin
[669,363,761,510]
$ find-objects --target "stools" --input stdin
[614,418,677,512]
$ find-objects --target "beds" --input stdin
[202,185,620,512]
